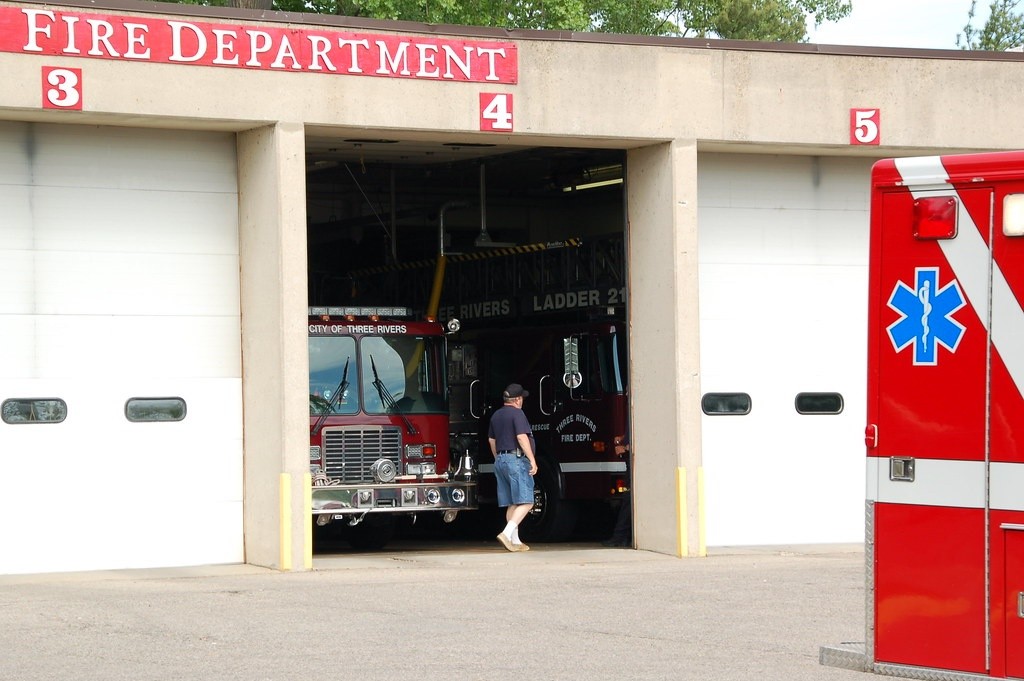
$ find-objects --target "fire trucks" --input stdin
[301,303,493,551]
[419,305,631,543]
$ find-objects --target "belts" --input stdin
[497,449,524,457]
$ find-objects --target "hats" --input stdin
[504,384,529,397]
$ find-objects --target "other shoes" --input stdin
[496,532,530,552]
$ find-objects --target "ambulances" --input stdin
[815,146,1024,681]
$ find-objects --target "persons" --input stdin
[488,384,538,551]
